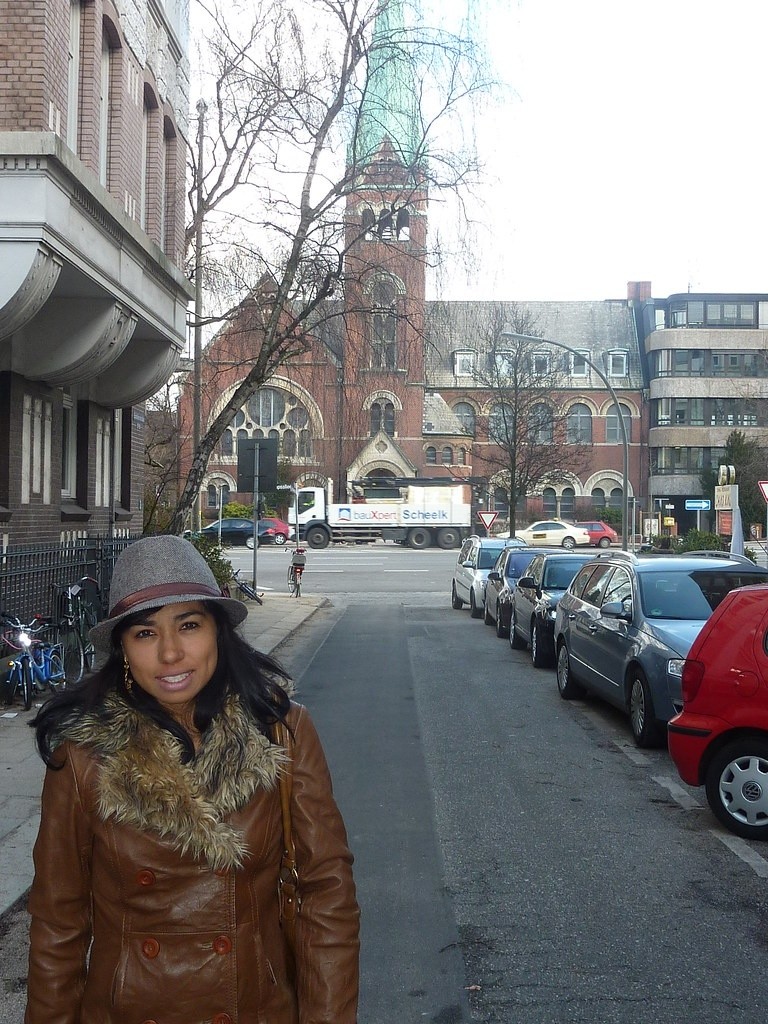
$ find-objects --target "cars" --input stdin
[183,518,275,550]
[258,518,289,545]
[509,553,610,668]
[495,518,590,549]
[484,546,574,638]
[668,582,768,840]
[568,520,618,548]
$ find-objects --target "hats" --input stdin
[89,534,249,654]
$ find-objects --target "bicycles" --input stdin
[51,576,98,686]
[0,611,66,709]
[228,569,264,605]
[284,546,307,598]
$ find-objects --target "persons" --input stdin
[23,535,361,1024]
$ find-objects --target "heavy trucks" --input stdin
[287,476,487,551]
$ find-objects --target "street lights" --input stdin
[500,332,628,547]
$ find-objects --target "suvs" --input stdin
[554,550,768,748]
[452,535,529,618]
